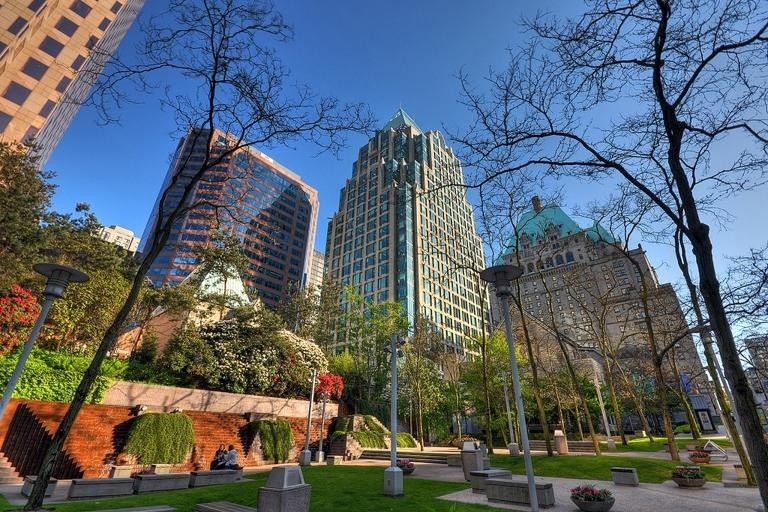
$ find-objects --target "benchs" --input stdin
[446,454,639,510]
[68,479,135,500]
[134,473,190,495]
[189,470,235,488]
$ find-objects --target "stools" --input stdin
[326,455,343,465]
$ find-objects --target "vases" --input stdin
[675,478,705,490]
[689,457,710,464]
[402,467,413,475]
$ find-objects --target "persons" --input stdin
[222,445,238,469]
[210,443,227,470]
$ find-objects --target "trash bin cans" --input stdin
[554,429,568,456]
[460,449,483,482]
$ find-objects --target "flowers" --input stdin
[397,457,414,467]
[691,450,710,457]
[671,465,706,478]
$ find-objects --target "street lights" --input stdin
[0,260,93,422]
[296,367,322,467]
[496,365,519,457]
[588,374,616,450]
[382,331,405,496]
[476,264,540,512]
[313,389,330,463]
[687,325,742,433]
